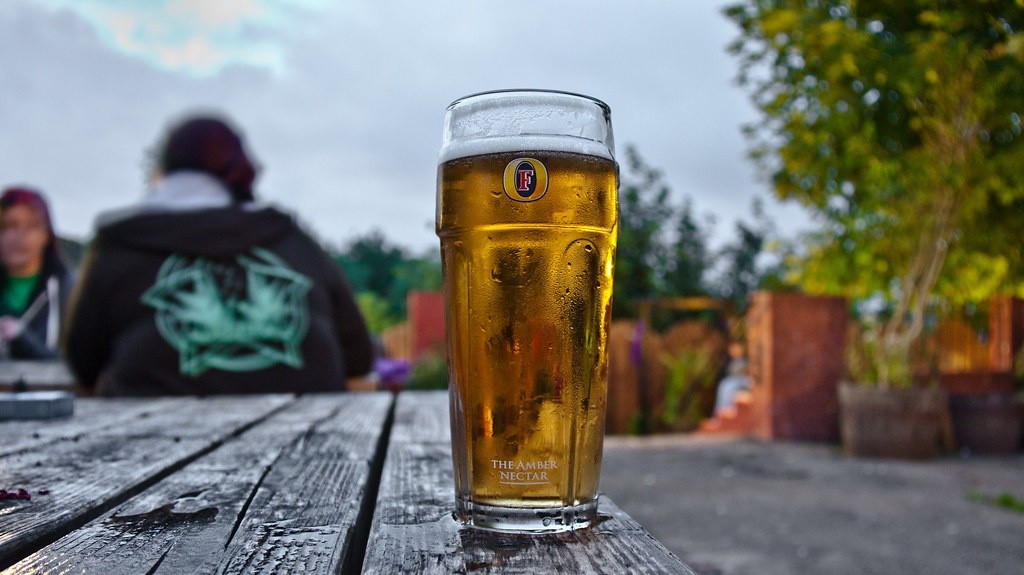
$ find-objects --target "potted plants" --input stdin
[837,321,940,459]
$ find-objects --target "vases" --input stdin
[952,393,1024,455]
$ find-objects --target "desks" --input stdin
[1,390,699,575]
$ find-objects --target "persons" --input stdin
[67,115,376,398]
[0,187,72,359]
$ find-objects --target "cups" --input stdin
[435,88,621,534]
